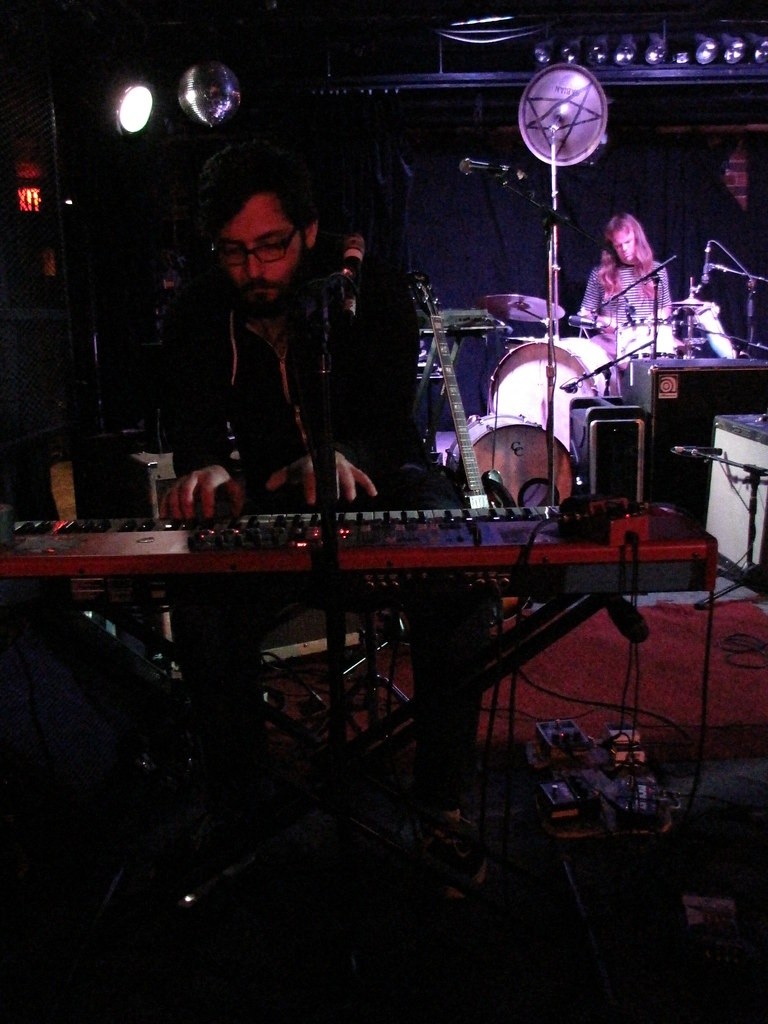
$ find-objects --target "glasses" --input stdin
[211,221,303,267]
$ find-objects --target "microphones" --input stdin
[699,262,710,285]
[457,157,517,175]
[336,230,366,317]
[676,444,721,455]
[564,382,578,393]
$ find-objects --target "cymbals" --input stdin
[662,296,705,308]
[477,293,565,323]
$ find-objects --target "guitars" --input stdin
[410,269,491,509]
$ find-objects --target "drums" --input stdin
[617,322,675,364]
[486,337,618,451]
[439,412,574,507]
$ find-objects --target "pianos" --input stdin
[1,501,722,602]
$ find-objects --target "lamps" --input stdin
[533,31,768,66]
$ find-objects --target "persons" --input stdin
[576,210,674,365]
[158,137,503,904]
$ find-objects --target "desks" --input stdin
[412,325,513,441]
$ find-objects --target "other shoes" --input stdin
[151,815,258,901]
[426,819,487,902]
